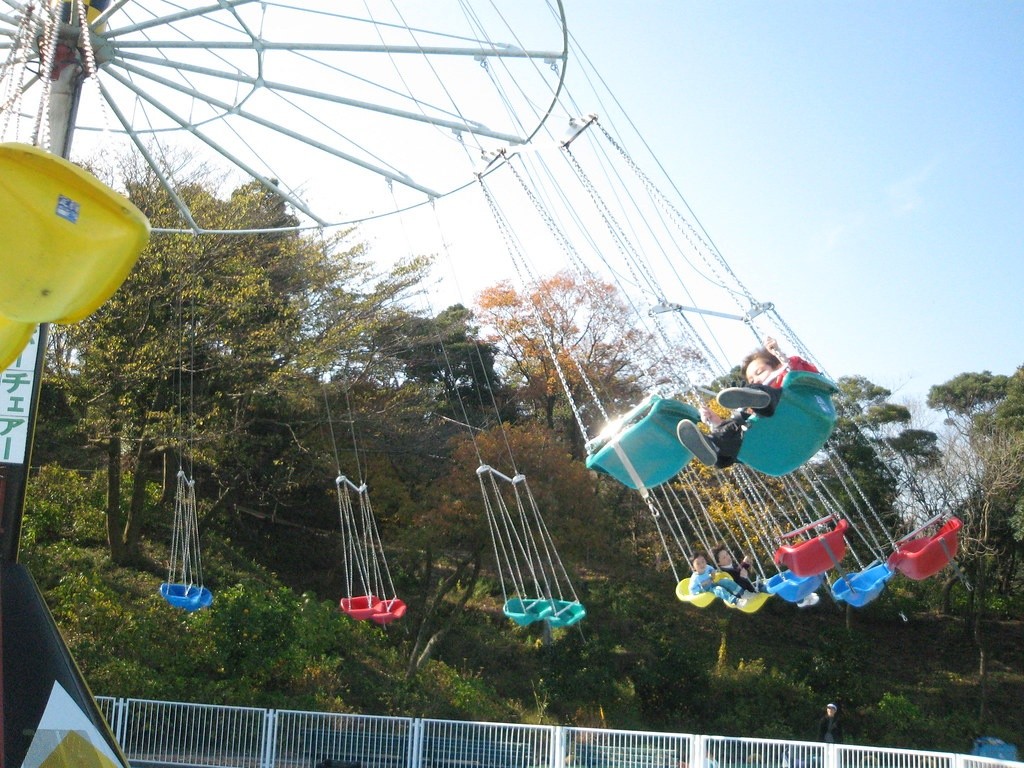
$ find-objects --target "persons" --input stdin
[689,551,754,606]
[677,336,818,469]
[817,703,843,744]
[710,545,819,607]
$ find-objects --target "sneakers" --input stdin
[736,589,756,607]
[797,592,820,607]
[676,419,719,468]
[715,387,771,410]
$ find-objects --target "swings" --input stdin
[397,200,588,629]
[295,228,407,625]
[158,231,216,610]
[364,0,962,611]
